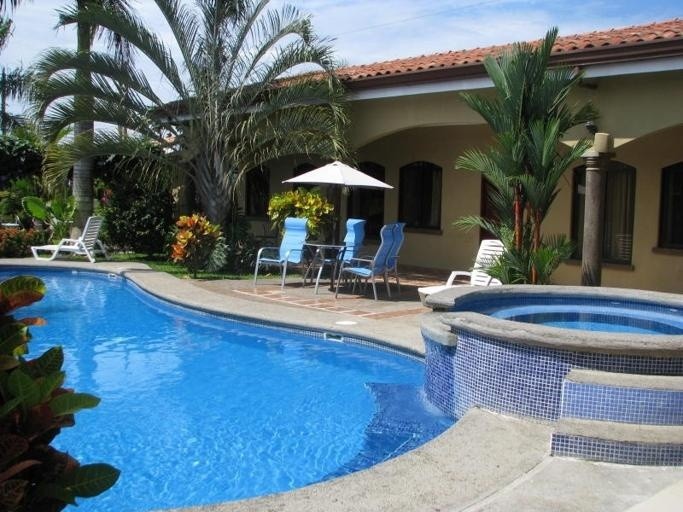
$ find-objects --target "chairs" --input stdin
[29,216,109,263]
[310,218,406,302]
[417,239,506,308]
[253,217,309,289]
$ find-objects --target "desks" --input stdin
[301,244,355,295]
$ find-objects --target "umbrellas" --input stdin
[281,158,396,244]
